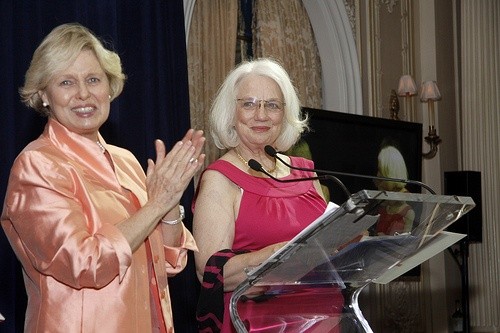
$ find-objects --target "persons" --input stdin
[191,59,344,333]
[0,23,208,333]
[373,146,415,235]
[285,140,329,204]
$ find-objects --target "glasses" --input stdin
[234,98,287,112]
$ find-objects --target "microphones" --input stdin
[264,145,437,194]
[249,159,352,199]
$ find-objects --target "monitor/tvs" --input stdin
[302,107,423,282]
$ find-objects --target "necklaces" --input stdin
[97,141,105,154]
[235,148,277,173]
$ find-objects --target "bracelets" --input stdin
[161,204,185,225]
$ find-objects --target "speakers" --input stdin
[444,170,482,244]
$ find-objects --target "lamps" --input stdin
[391,75,442,159]
